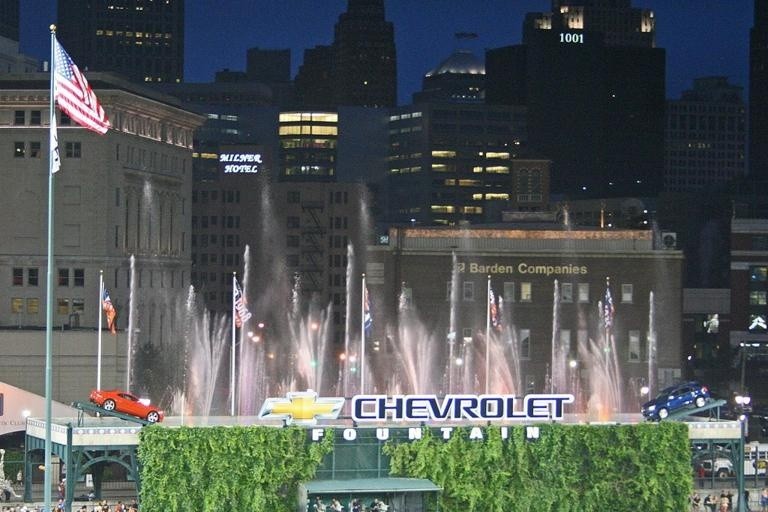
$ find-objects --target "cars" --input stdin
[640,382,711,420]
[89,389,165,424]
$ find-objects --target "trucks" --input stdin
[702,441,768,482]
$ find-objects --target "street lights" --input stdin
[734,394,751,512]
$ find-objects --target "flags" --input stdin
[605,285,616,328]
[102,283,118,338]
[365,284,373,334]
[235,279,250,328]
[55,34,111,141]
[490,284,503,331]
